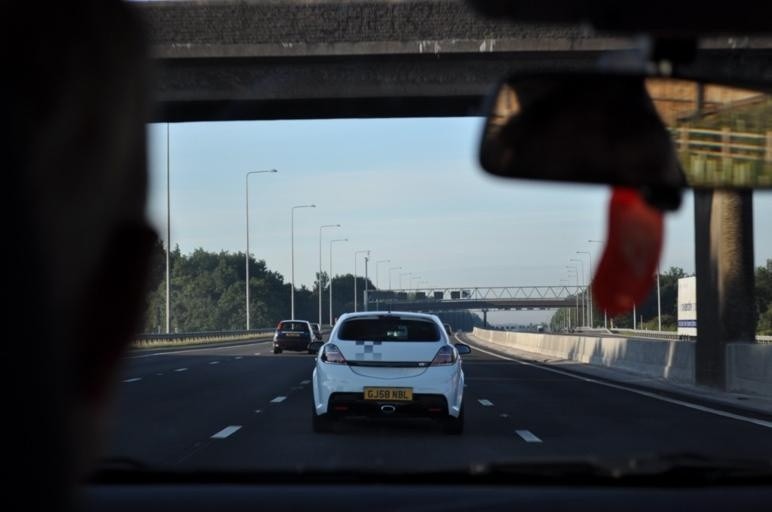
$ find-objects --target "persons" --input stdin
[0,0,169,485]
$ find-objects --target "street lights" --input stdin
[354,250,429,310]
[319,224,349,330]
[561,238,662,331]
[290,205,316,329]
[246,170,278,330]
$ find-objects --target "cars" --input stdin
[272,320,322,353]
[307,311,470,434]
